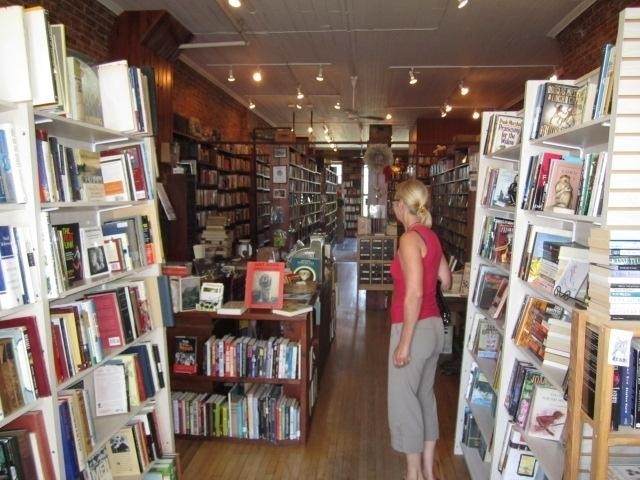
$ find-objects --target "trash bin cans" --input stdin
[441,326,454,354]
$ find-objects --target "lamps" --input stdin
[216,54,493,117]
[447,0,470,10]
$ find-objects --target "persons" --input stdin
[388,178,452,480]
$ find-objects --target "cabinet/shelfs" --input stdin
[366,122,394,233]
[431,277,468,374]
[489,8,640,479]
[355,233,400,305]
[178,138,270,248]
[171,248,338,446]
[450,8,576,479]
[0,29,71,480]
[410,161,480,270]
[271,144,340,254]
[21,37,180,480]
[562,309,640,479]
[341,178,362,238]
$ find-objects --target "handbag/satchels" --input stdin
[435,280,450,327]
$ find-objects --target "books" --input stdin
[355,152,477,285]
[1,6,182,480]
[458,44,639,479]
[166,115,364,444]
